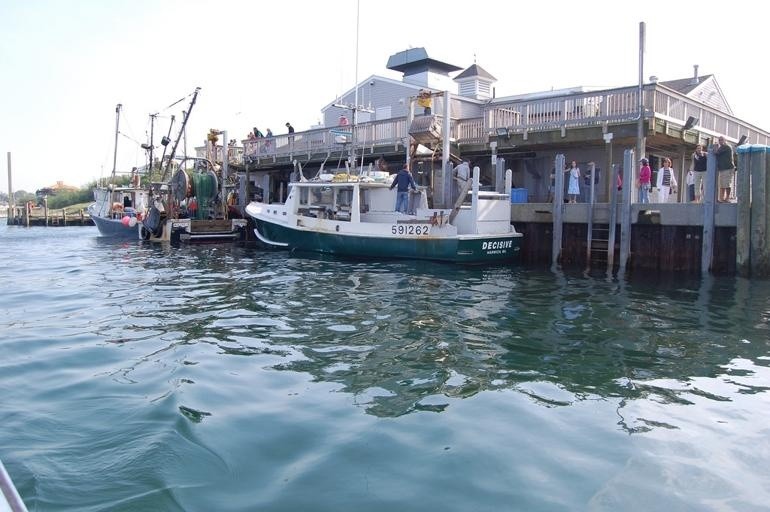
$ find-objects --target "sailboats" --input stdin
[87,86,248,245]
[244,0,528,266]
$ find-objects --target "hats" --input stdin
[638,158,649,163]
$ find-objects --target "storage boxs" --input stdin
[510,187,529,204]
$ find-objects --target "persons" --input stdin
[616,174,623,190]
[563,160,581,203]
[338,114,348,125]
[285,122,294,144]
[656,156,677,202]
[245,126,273,154]
[585,160,596,202]
[684,169,694,202]
[691,144,708,203]
[388,163,417,214]
[453,158,470,193]
[27,200,34,216]
[547,162,568,203]
[637,156,652,204]
[714,136,734,203]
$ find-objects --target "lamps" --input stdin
[736,134,751,148]
[496,126,511,138]
[684,114,700,134]
